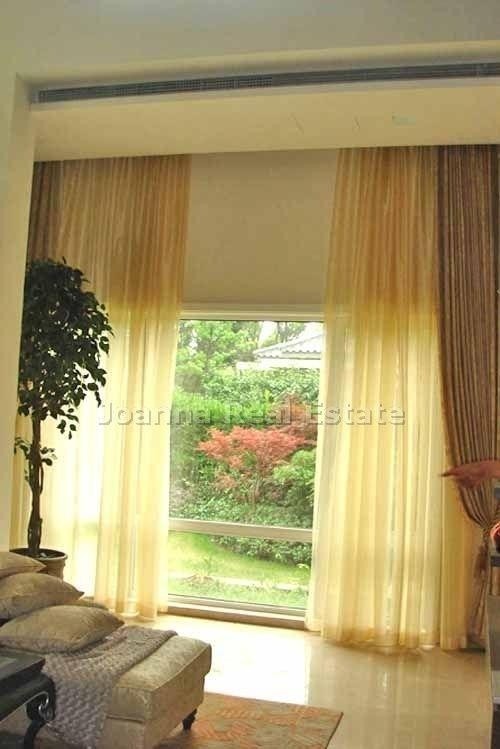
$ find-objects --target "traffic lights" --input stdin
[0,551,125,654]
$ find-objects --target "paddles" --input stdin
[0,692,344,748]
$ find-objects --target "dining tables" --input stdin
[13,256,116,580]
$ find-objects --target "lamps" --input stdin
[0,625,211,748]
